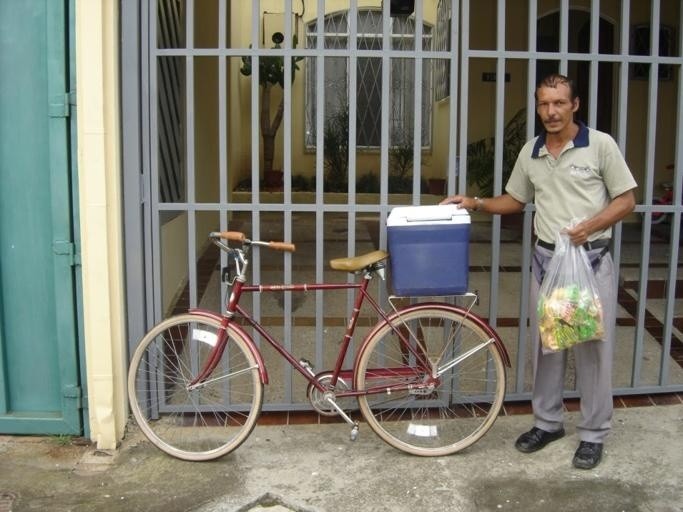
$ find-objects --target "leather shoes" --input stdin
[513,425,566,453]
[572,440,603,470]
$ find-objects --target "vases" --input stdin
[427,178,445,196]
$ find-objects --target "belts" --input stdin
[537,238,607,250]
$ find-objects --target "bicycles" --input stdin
[639,164,673,224]
[126,231,511,462]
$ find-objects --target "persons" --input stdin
[440,73,641,466]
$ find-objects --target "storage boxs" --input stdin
[384,201,471,295]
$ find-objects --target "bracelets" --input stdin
[469,192,486,213]
